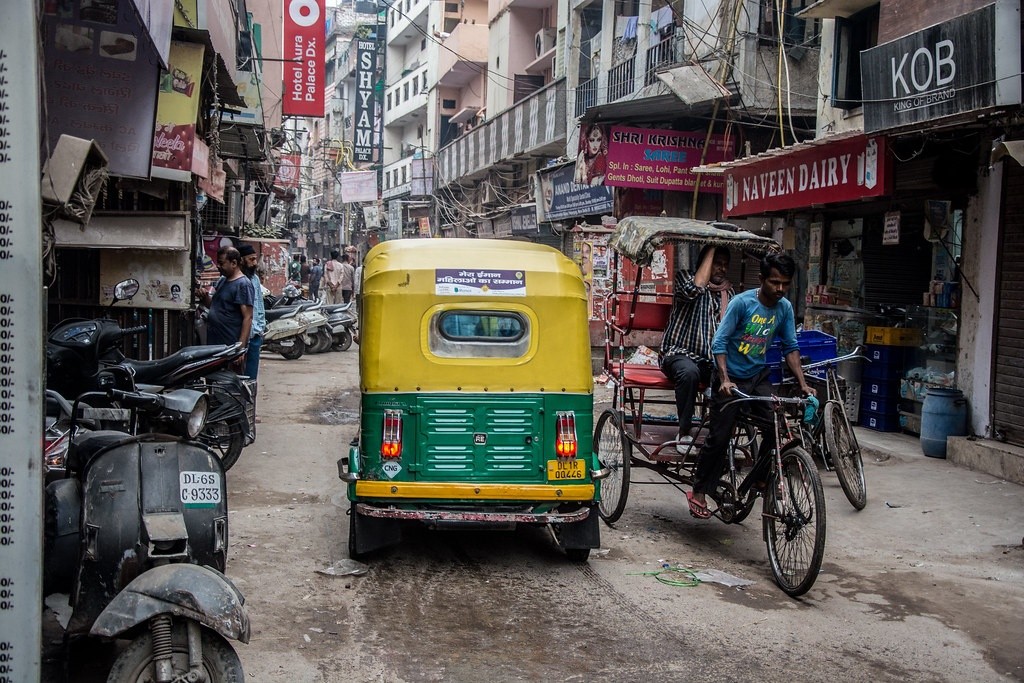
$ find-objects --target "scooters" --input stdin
[262,283,358,361]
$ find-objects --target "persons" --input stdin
[574,124,608,186]
[593,246,606,257]
[197,247,253,374]
[685,254,817,518]
[288,255,301,287]
[216,246,265,379]
[659,245,737,455]
[324,251,343,303]
[300,256,311,298]
[580,243,591,274]
[353,258,364,330]
[342,255,355,303]
[308,257,323,299]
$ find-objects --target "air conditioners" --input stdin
[535,27,557,59]
[203,185,234,231]
[529,173,536,201]
[482,179,496,204]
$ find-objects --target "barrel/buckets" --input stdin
[920,385,967,458]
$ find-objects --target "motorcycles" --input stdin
[42,279,257,683]
[339,238,601,565]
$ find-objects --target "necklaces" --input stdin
[756,290,758,297]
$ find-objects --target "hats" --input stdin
[236,245,256,257]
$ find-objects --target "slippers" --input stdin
[686,490,711,518]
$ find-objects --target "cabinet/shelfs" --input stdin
[901,306,960,432]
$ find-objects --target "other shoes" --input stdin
[676,434,696,456]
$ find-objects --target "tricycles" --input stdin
[734,344,873,510]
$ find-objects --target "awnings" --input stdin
[220,121,264,158]
[578,83,741,121]
[691,128,865,173]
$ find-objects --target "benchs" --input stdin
[604,291,671,387]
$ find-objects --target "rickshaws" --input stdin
[594,215,827,596]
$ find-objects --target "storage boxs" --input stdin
[858,326,923,432]
[764,328,839,383]
[924,281,960,307]
